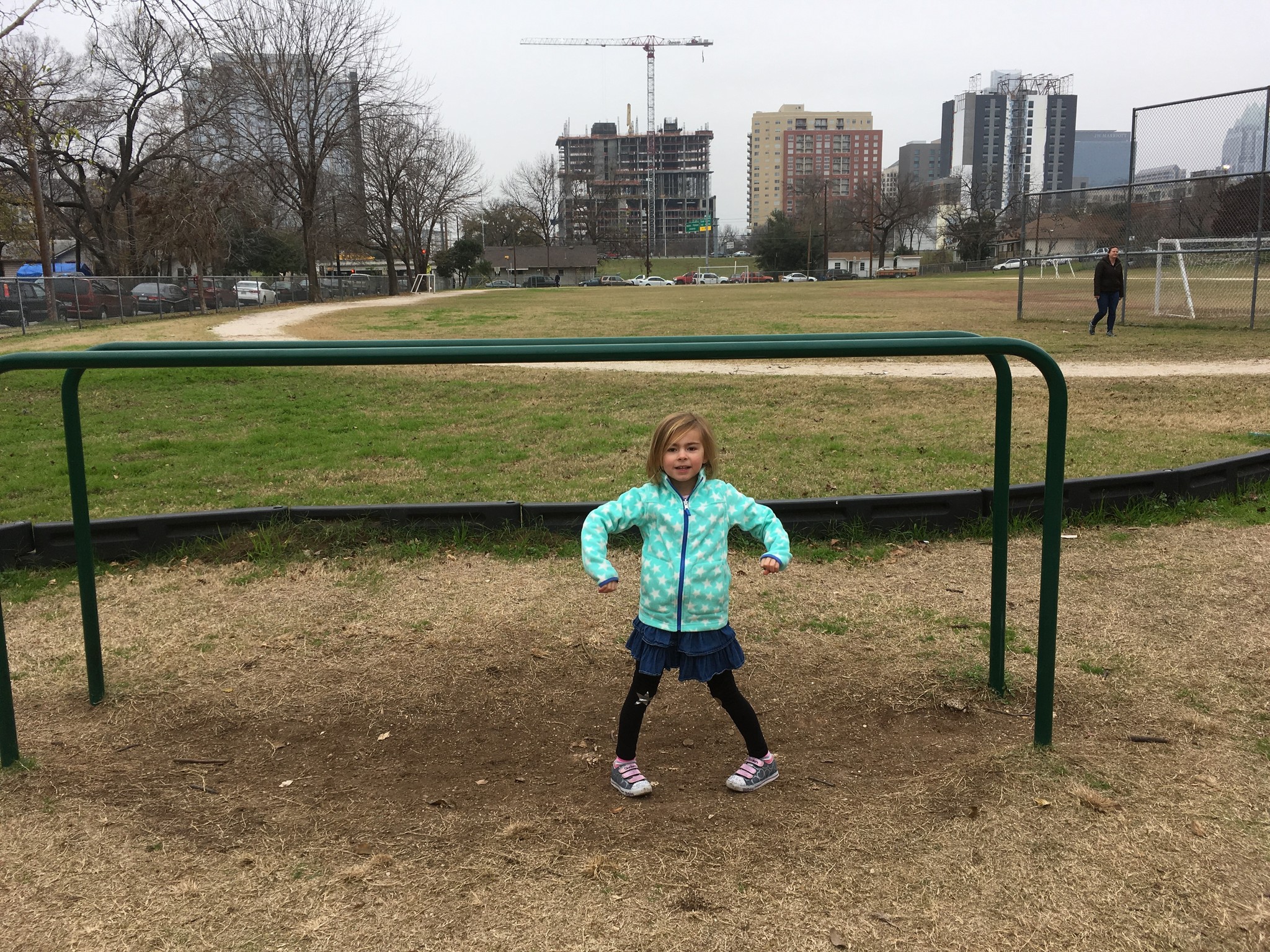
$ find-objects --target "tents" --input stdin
[17,263,93,285]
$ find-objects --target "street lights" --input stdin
[469,179,484,250]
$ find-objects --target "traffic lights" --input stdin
[505,256,509,260]
[351,270,355,273]
[422,249,427,254]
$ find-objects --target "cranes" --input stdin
[521,37,714,254]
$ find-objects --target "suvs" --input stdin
[0,272,140,328]
[528,276,560,287]
[600,276,631,286]
[823,269,859,281]
[691,272,729,284]
[728,272,774,284]
[673,272,698,285]
[181,278,238,310]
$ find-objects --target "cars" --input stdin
[1128,246,1158,262]
[782,273,817,282]
[233,280,352,305]
[733,251,749,257]
[992,259,1028,270]
[484,280,521,288]
[639,276,675,286]
[578,278,602,287]
[129,282,195,314]
[1040,255,1072,267]
[709,252,729,258]
[373,275,407,294]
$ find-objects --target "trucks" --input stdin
[346,274,377,296]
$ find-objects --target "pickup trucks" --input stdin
[1082,248,1124,261]
[627,274,658,286]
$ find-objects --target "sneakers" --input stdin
[610,760,652,797]
[1106,330,1117,337]
[726,753,780,792]
[1089,321,1096,335]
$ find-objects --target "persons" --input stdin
[453,278,456,289]
[554,273,561,288]
[581,415,792,797]
[1088,246,1124,338]
